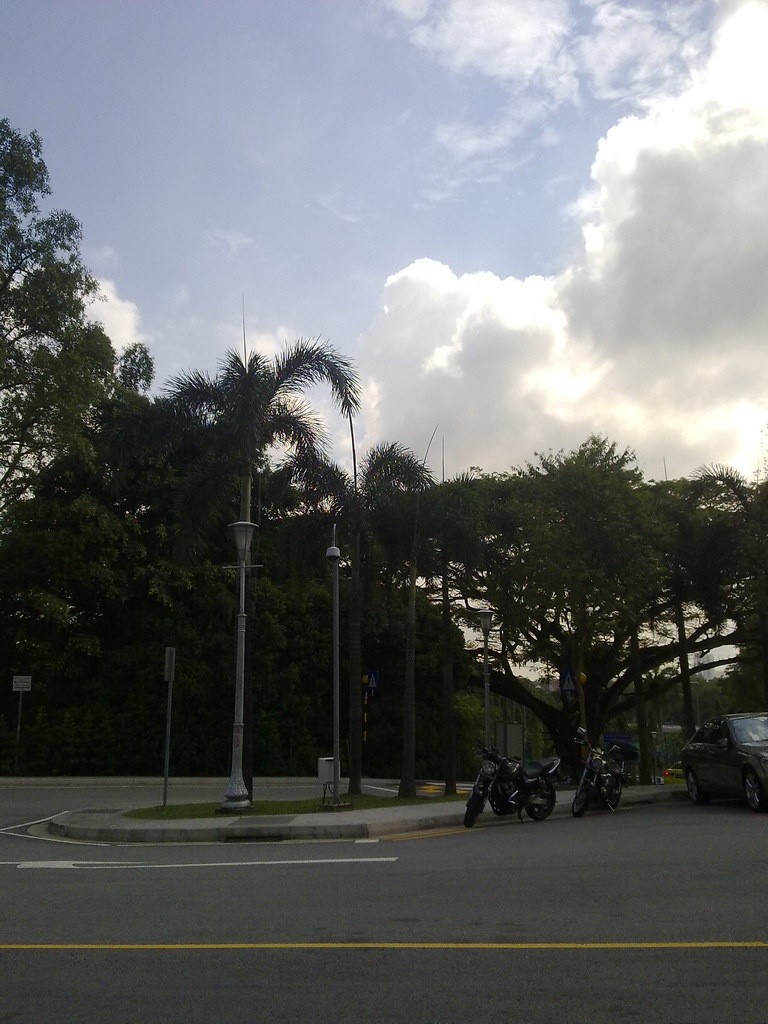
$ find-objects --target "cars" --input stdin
[680,711,768,813]
[666,761,687,776]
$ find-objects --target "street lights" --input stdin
[219,520,261,813]
[474,608,497,749]
[324,546,350,806]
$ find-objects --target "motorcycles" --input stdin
[571,726,626,816]
[462,742,561,829]
